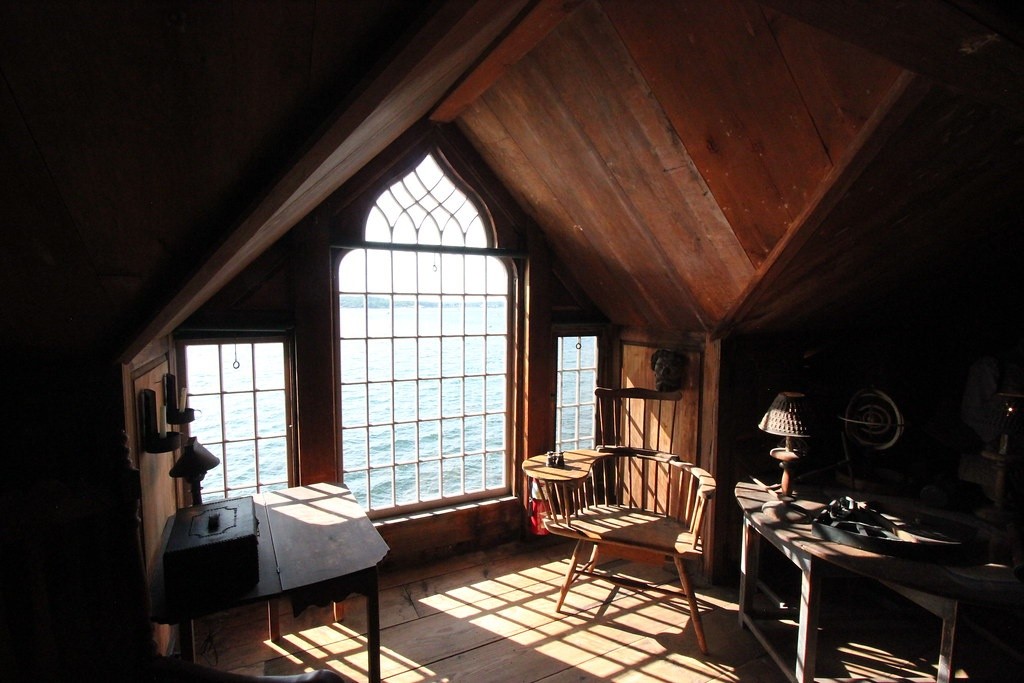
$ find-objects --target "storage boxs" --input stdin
[163,495,261,595]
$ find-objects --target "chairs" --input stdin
[521,387,721,656]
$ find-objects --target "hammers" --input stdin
[831,521,883,538]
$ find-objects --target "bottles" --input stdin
[546,450,565,468]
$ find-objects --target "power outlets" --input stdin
[169,638,180,661]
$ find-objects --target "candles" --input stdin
[178,384,188,416]
[158,403,167,440]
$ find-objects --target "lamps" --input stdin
[757,391,819,508]
[169,437,219,509]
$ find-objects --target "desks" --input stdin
[151,481,385,683]
[734,468,1024,683]
